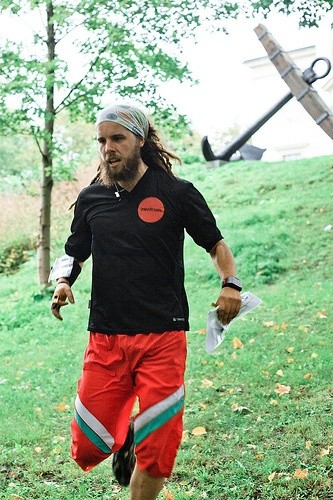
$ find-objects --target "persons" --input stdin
[50,104,242,500]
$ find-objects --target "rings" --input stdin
[53,295,59,303]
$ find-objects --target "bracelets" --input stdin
[56,281,70,288]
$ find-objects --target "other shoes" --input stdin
[112,416,137,487]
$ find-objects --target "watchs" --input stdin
[221,275,242,292]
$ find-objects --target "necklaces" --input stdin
[113,181,138,198]
